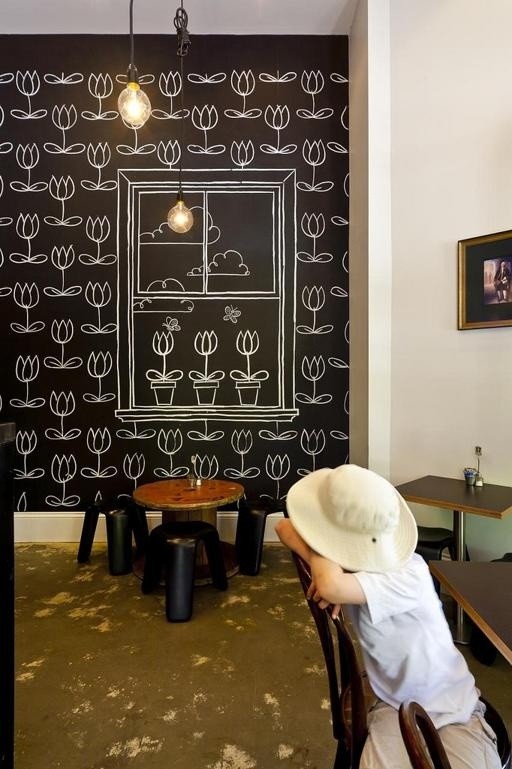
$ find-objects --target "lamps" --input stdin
[115,1,198,232]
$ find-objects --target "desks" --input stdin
[132,474,245,586]
[388,475,512,668]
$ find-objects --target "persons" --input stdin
[275,462,503,769]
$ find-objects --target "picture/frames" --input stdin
[453,226,509,332]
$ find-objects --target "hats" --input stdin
[286,463,418,575]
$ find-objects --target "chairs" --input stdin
[289,548,511,769]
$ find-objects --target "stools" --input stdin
[76,496,286,625]
